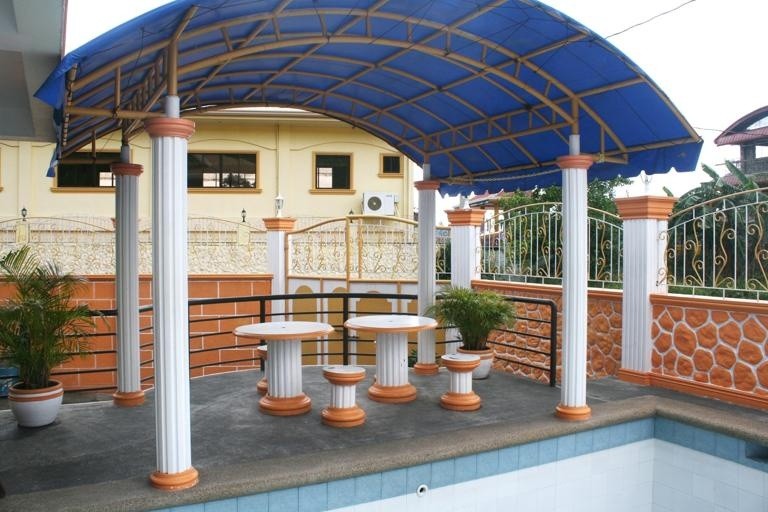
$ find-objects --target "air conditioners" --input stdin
[362,192,395,217]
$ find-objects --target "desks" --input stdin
[233,314,438,417]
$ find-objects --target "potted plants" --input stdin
[422,285,520,379]
[0,245,112,428]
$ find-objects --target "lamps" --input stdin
[21,208,27,222]
[241,208,247,222]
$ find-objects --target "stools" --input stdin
[255,344,481,428]
